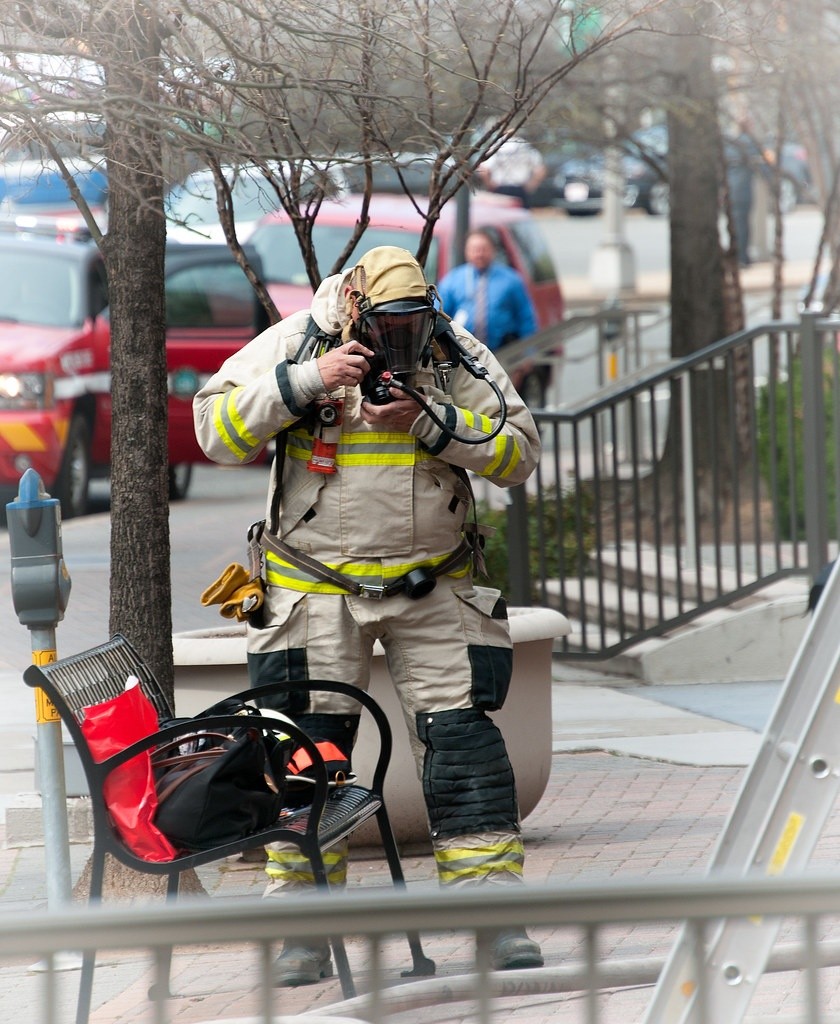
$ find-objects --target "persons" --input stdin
[193,246,543,987]
[721,116,761,266]
[431,229,537,404]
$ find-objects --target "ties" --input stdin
[477,274,487,342]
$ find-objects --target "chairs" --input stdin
[23,632,437,1024]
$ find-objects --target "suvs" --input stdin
[0,217,272,519]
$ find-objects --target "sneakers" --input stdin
[474,923,543,968]
[272,935,334,988]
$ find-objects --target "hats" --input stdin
[347,245,430,316]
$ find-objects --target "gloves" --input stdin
[200,563,264,622]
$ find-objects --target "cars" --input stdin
[444,118,560,205]
[164,151,464,247]
[555,125,809,217]
[1,110,108,173]
[1,49,107,115]
[1,156,110,235]
[212,192,565,408]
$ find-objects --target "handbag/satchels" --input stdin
[85,675,175,867]
[149,698,352,853]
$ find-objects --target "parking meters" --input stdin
[5,468,75,975]
